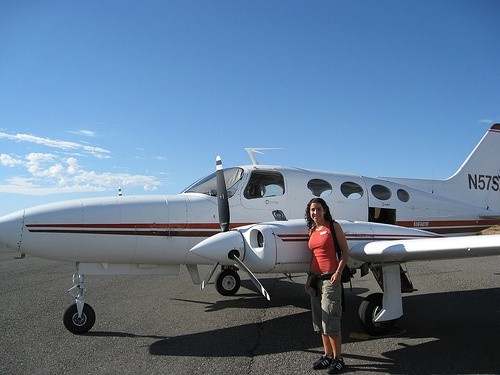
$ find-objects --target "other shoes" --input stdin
[312,354,333,370]
[326,357,346,374]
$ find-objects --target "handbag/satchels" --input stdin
[341,264,351,283]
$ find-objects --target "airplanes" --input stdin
[0,123,499,336]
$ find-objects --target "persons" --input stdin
[305,198,349,375]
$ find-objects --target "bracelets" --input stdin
[336,270,342,274]
